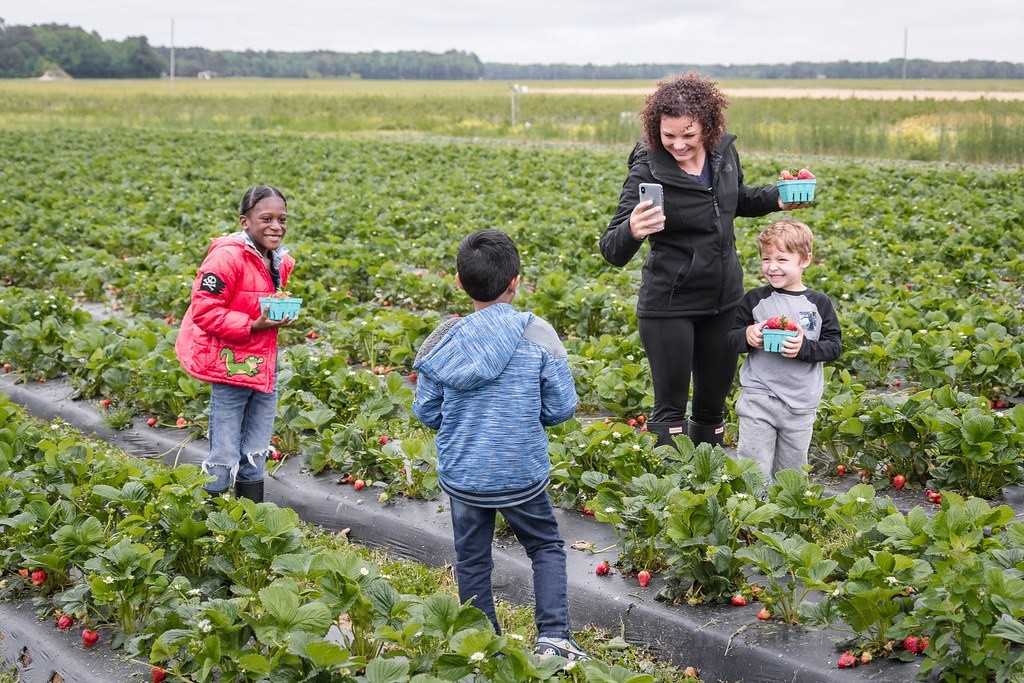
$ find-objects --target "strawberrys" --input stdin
[729,587,774,619]
[837,464,949,506]
[595,561,651,589]
[777,169,816,180]
[2,254,648,490]
[20,569,168,683]
[763,315,797,331]
[838,653,874,670]
[902,635,930,654]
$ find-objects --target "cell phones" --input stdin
[639,182,664,229]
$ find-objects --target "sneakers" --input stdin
[531,635,593,675]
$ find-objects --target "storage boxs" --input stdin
[257,297,302,322]
[761,328,801,354]
[777,179,817,203]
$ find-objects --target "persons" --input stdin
[724,219,842,506]
[600,72,812,475]
[173,184,297,531]
[410,227,594,669]
